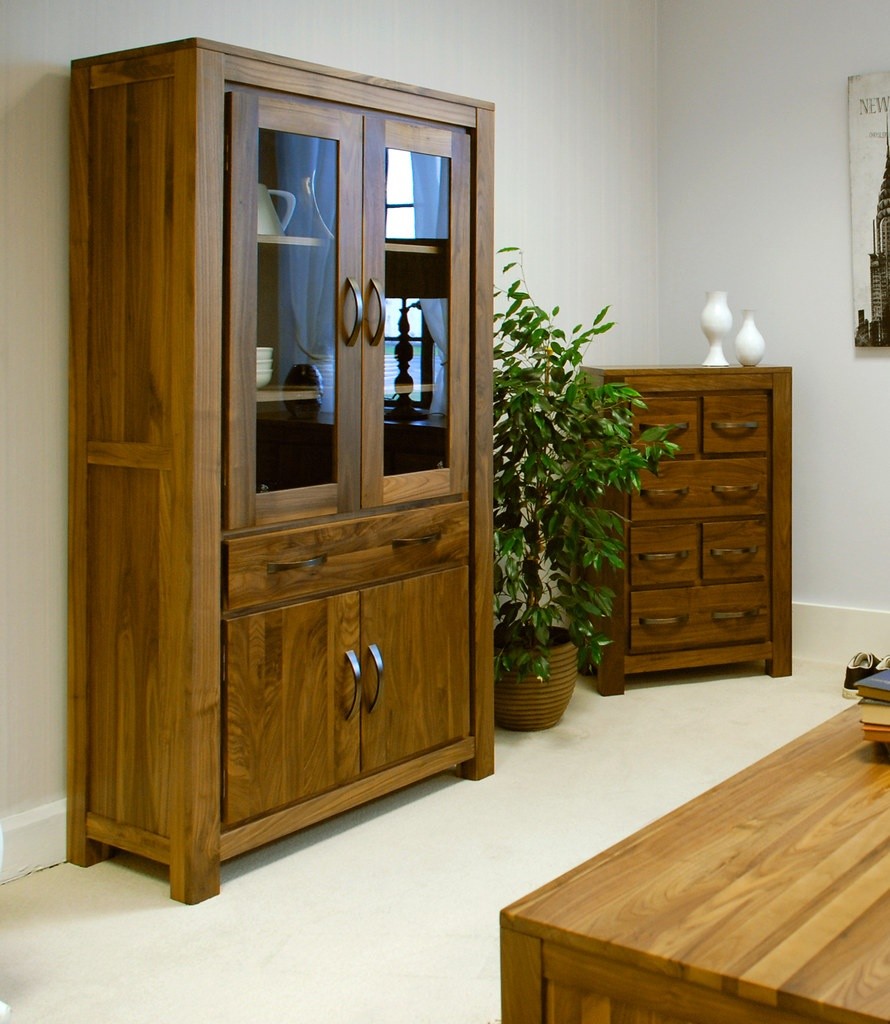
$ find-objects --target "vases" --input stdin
[734,309,765,367]
[698,291,733,367]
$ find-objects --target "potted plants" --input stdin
[492,247,690,733]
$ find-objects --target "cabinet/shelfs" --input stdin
[572,366,793,697]
[66,38,495,902]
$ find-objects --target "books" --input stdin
[853,667,890,754]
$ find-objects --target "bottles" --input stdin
[734,309,765,367]
[697,290,734,367]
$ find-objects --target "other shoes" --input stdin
[843,651,884,699]
[875,654,890,676]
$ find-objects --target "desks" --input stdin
[500,693,890,1024]
